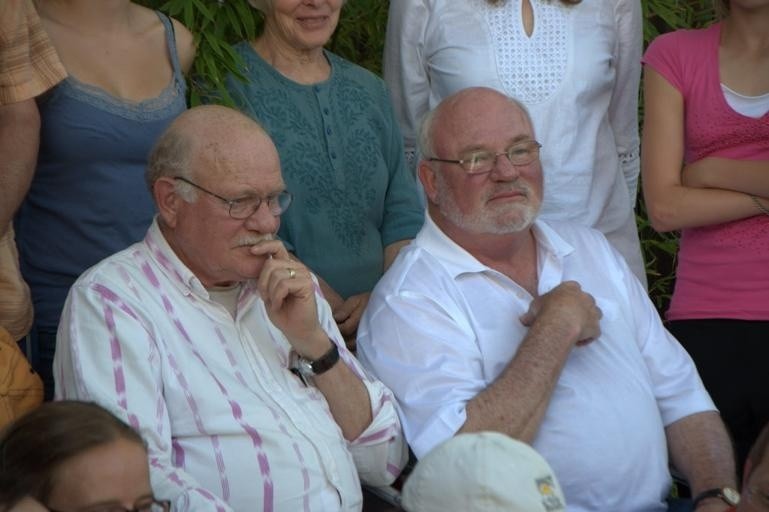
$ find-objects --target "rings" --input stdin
[287,269,296,278]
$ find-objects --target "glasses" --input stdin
[171,175,294,221]
[428,139,542,174]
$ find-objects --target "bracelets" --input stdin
[750,194,769,217]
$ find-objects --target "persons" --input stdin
[1,399,154,511]
[357,87,739,512]
[1,0,69,431]
[641,1,769,491]
[53,105,407,512]
[14,2,427,405]
[383,2,648,295]
[740,424,769,512]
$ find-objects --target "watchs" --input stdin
[299,338,340,376]
[691,486,741,510]
